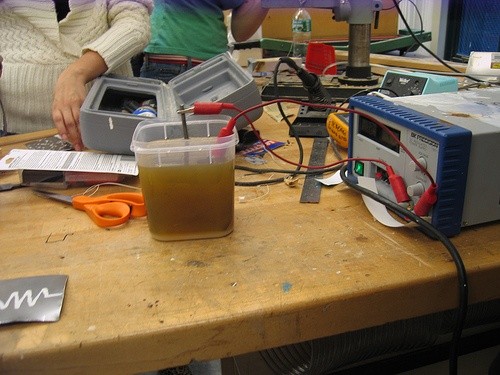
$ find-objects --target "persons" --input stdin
[0,0,155,151]
[139,0,270,85]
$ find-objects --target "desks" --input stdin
[0,76,499,375]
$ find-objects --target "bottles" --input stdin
[291,7,312,65]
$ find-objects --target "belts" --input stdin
[143,54,204,66]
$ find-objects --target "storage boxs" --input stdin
[78,51,263,154]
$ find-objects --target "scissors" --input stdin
[30,186,147,232]
